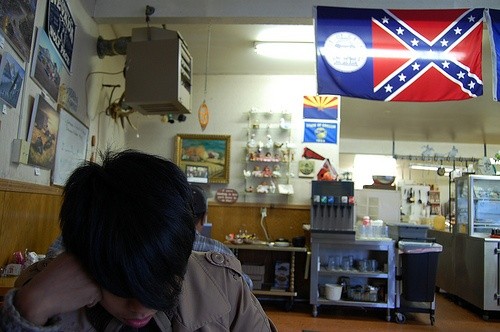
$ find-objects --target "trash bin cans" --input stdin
[398,242,443,302]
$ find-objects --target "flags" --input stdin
[315,4,485,102]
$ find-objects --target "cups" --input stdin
[325,283,343,301]
[328,256,376,273]
[359,224,391,238]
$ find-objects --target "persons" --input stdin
[43,232,65,259]
[187,182,255,293]
[1,143,278,332]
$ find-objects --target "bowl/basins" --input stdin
[231,239,244,244]
[372,176,395,185]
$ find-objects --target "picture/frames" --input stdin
[45,0,76,69]
[175,133,231,184]
[30,28,62,104]
[0,0,37,61]
[0,53,26,108]
[52,105,89,187]
[27,93,59,171]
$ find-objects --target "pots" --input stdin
[437,160,445,176]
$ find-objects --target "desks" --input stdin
[224,243,306,306]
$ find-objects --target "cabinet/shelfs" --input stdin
[306,233,397,321]
[453,175,500,233]
[244,111,292,196]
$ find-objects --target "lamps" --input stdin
[410,163,466,171]
[253,40,314,51]
